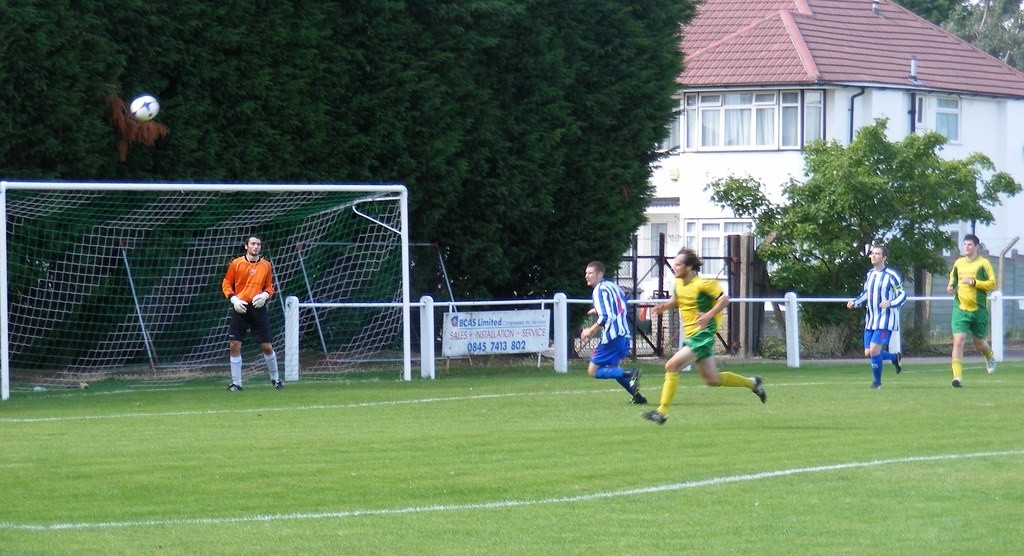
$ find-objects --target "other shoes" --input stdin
[226,384,244,391]
[272,379,283,390]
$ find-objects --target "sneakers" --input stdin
[639,410,668,425]
[951,378,963,389]
[752,376,767,404]
[628,368,642,395]
[891,352,902,374]
[627,397,648,405]
[985,350,996,374]
[870,381,882,390]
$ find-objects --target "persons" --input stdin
[846,245,906,389]
[581,261,647,405]
[947,234,997,388]
[641,249,766,425]
[221,236,285,390]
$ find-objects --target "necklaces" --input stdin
[247,256,259,273]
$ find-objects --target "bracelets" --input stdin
[972,280,973,284]
[974,280,976,286]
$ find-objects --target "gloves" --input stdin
[252,292,269,308]
[229,296,249,314]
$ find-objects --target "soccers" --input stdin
[129,95,161,120]
